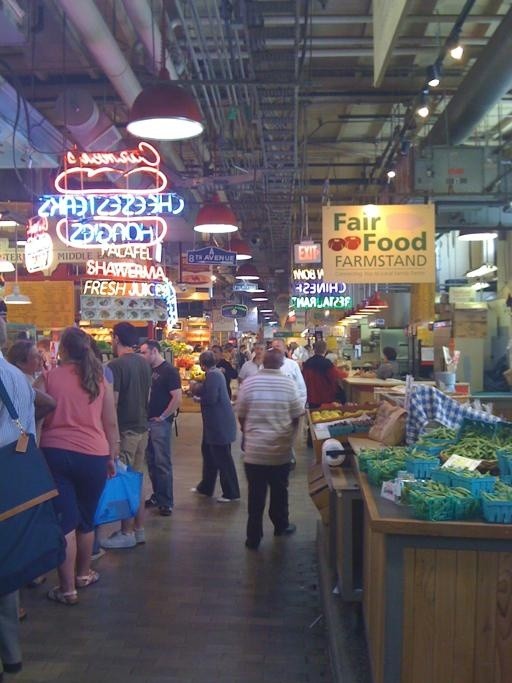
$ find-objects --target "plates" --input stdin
[301,419,366,612]
[167,317,213,346]
[429,337,487,392]
[345,441,512,682]
[345,376,404,409]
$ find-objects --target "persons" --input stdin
[368,346,402,379]
[211,338,348,487]
[100,322,152,547]
[187,350,242,503]
[31,328,119,606]
[140,340,182,516]
[3,356,57,671]
[9,340,42,385]
[237,347,304,548]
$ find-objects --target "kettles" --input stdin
[358,426,512,516]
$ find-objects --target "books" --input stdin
[422,282,492,337]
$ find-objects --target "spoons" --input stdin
[344,236,361,250]
[328,238,345,251]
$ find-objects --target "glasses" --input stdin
[458,228,500,242]
[332,275,389,327]
[123,1,206,143]
[427,60,440,88]
[188,150,282,331]
[3,218,32,305]
[464,238,499,280]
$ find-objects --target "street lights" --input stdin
[91,459,144,528]
[0,433,69,600]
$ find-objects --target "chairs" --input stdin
[274,523,296,537]
[245,537,261,550]
[47,585,78,605]
[189,486,216,497]
[98,527,147,549]
[75,569,101,588]
[217,495,242,503]
[4,660,24,674]
[27,577,47,589]
[144,493,173,517]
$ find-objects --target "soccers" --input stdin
[312,410,341,422]
